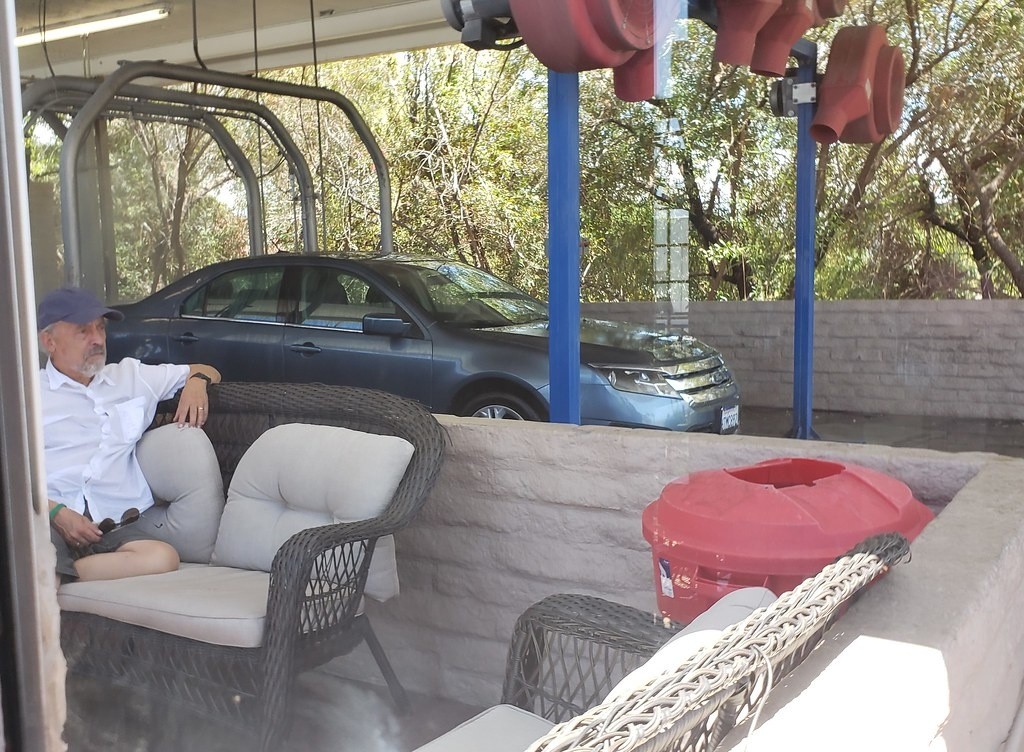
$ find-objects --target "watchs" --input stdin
[189,372,211,384]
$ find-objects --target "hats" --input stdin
[37,286,124,329]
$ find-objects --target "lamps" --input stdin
[9,5,172,49]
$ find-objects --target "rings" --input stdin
[198,407,204,410]
[77,542,80,546]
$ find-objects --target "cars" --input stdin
[100,253,742,438]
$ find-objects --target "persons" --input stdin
[34,286,222,593]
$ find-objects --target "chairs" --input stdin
[314,281,348,318]
[366,282,394,309]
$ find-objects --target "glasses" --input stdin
[98,508,163,536]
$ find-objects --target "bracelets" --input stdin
[49,503,67,520]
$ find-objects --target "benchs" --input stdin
[61,380,447,752]
[213,283,282,303]
[408,532,950,751]
